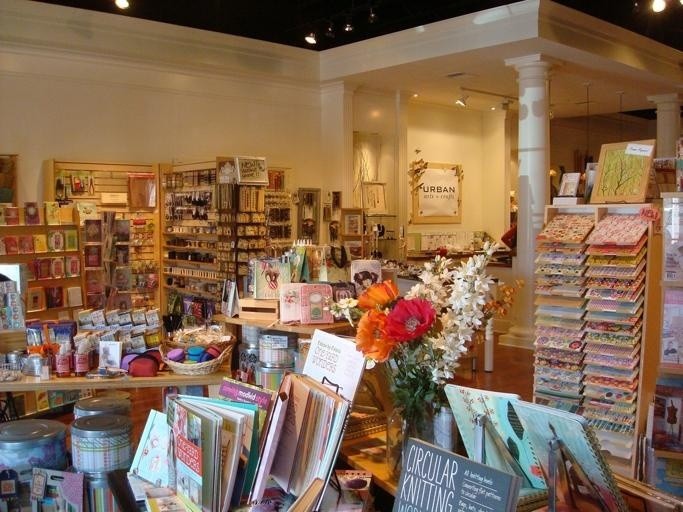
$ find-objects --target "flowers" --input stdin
[356,240,524,424]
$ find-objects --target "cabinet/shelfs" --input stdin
[531,205,663,480]
[162,161,217,298]
[0,207,93,421]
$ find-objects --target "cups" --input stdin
[165,224,218,264]
[309,292,323,319]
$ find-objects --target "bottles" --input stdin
[237,332,296,391]
[54,338,94,378]
[0,350,53,381]
[385,228,396,240]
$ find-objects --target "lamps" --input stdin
[456,95,469,107]
[304,8,375,44]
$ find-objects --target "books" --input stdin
[129,328,367,512]
[392,385,629,512]
[534,212,649,458]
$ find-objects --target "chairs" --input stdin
[340,208,373,260]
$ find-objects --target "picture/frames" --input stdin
[234,156,269,185]
[590,139,656,203]
[412,161,462,224]
[265,170,285,192]
[216,156,234,185]
[360,181,393,214]
[298,188,321,245]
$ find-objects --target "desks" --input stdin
[1,369,231,392]
[214,311,360,339]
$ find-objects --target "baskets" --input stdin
[159,314,236,375]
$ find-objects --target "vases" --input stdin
[386,407,407,485]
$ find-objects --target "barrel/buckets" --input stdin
[71,414,132,477]
[74,390,131,419]
[0,419,66,482]
